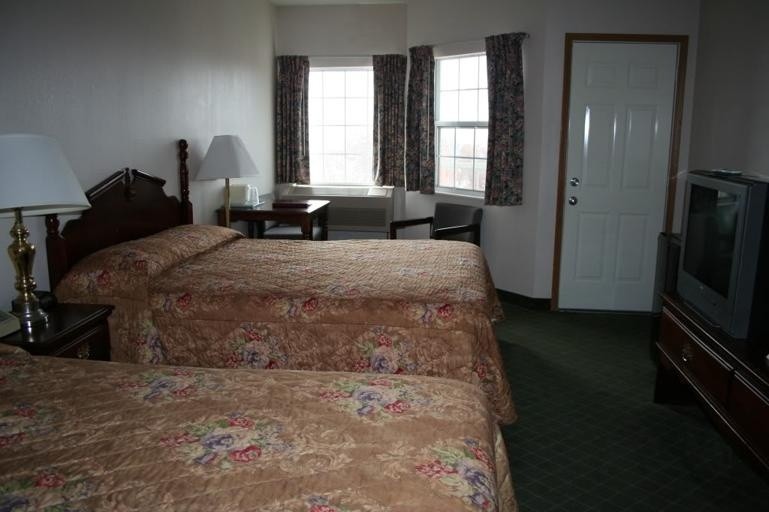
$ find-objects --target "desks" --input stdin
[213,197,331,240]
[650,288,769,474]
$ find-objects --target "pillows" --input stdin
[0,342,33,379]
[54,222,244,300]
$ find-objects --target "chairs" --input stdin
[256,190,322,240]
[390,202,483,247]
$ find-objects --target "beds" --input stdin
[0,340,519,511]
[46,139,518,427]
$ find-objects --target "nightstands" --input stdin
[0,298,116,360]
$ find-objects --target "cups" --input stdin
[244,184,259,202]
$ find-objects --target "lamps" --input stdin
[195,134,258,227]
[0,132,92,329]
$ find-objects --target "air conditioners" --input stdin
[276,182,395,232]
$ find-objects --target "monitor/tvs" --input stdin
[677,170,769,339]
